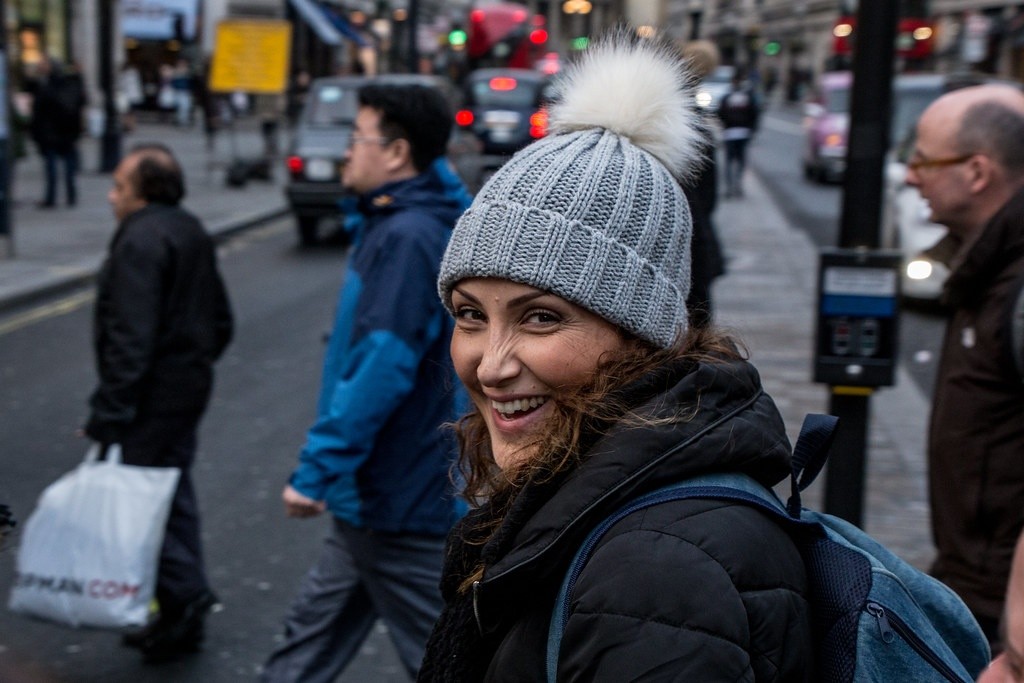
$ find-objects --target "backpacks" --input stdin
[545,414,993,683]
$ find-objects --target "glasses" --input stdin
[898,149,982,170]
[344,132,396,150]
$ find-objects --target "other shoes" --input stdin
[120,599,226,674]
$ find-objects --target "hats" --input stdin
[437,19,724,353]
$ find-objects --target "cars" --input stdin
[457,69,548,157]
[883,71,963,304]
[281,75,463,246]
[692,63,739,115]
[802,71,856,181]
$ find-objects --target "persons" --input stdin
[84,144,237,663]
[902,85,1024,682]
[252,41,809,683]
[717,67,761,195]
[29,51,89,209]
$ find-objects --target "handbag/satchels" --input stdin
[7,442,183,635]
